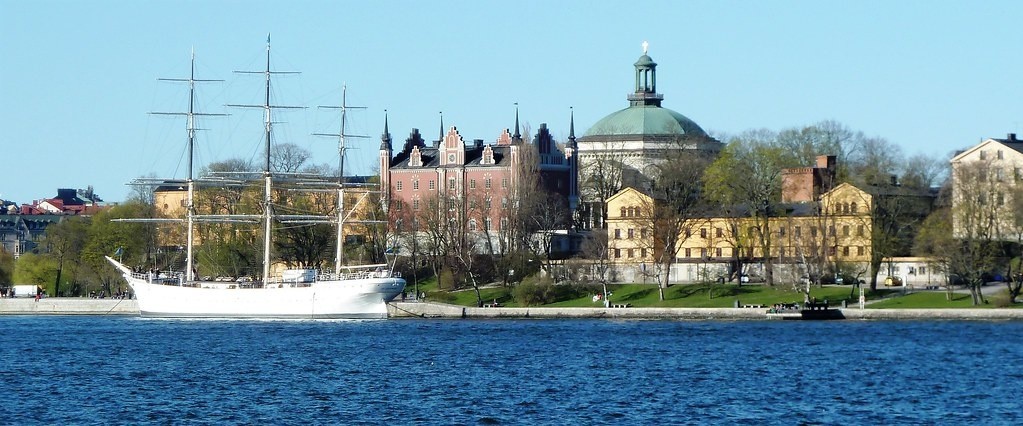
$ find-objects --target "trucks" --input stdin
[12,284,42,298]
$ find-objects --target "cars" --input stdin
[885,276,902,286]
[801,273,835,285]
[835,275,856,285]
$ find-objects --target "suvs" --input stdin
[741,274,764,283]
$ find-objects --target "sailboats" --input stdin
[103,33,406,319]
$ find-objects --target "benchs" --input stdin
[483,304,494,307]
[742,303,764,308]
[611,303,630,308]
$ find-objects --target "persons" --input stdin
[401,289,426,302]
